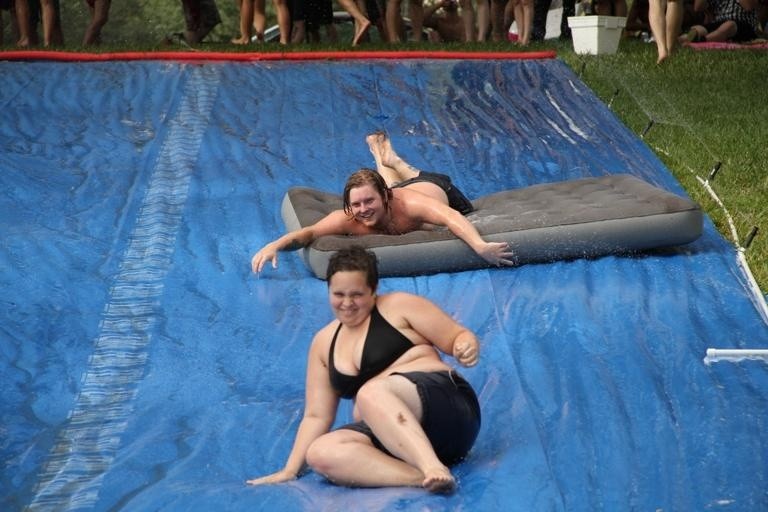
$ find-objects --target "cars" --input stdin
[250,9,440,44]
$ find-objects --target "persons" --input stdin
[13,0,112,51]
[246,243,481,496]
[626,1,768,63]
[231,0,629,47]
[251,130,515,273]
